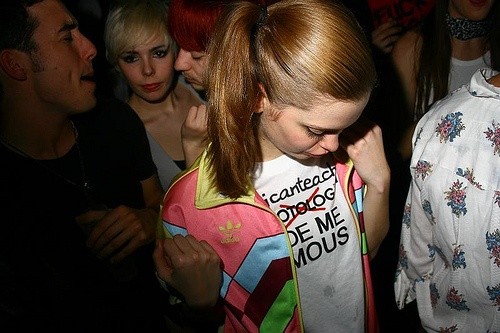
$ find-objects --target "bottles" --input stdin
[412,71,444,123]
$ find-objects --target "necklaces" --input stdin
[1,120,92,194]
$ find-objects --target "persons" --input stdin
[153,0,391,333]
[168,0,266,168]
[0,0,166,333]
[355,0,500,333]
[105,1,209,169]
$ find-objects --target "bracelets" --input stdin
[168,290,226,332]
